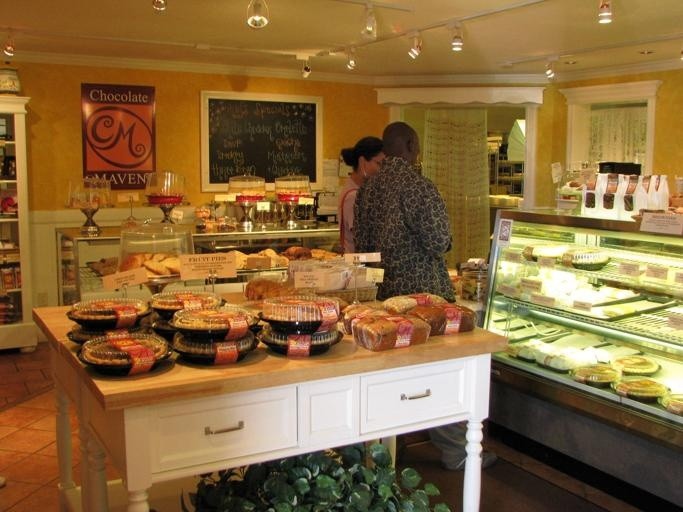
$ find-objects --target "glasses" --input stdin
[370,157,382,167]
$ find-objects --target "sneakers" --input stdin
[446,452,497,470]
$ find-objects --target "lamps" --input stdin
[595,1,614,24]
[298,4,467,80]
[544,56,556,80]
[150,1,168,16]
[1,29,16,56]
[245,1,271,29]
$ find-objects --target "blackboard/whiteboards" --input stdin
[200,90,324,192]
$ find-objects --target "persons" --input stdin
[337,134,385,256]
[350,121,500,472]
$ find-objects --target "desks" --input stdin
[32,285,508,512]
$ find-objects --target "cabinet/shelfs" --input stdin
[487,218,682,426]
[52,222,340,306]
[0,94,40,354]
[484,140,523,198]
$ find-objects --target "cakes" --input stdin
[569,246,610,269]
[610,377,666,400]
[658,394,682,411]
[64,299,172,375]
[150,288,260,365]
[262,331,344,356]
[573,364,620,384]
[259,294,340,336]
[614,354,659,375]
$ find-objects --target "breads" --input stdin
[246,282,295,301]
[120,246,338,276]
[342,292,478,350]
